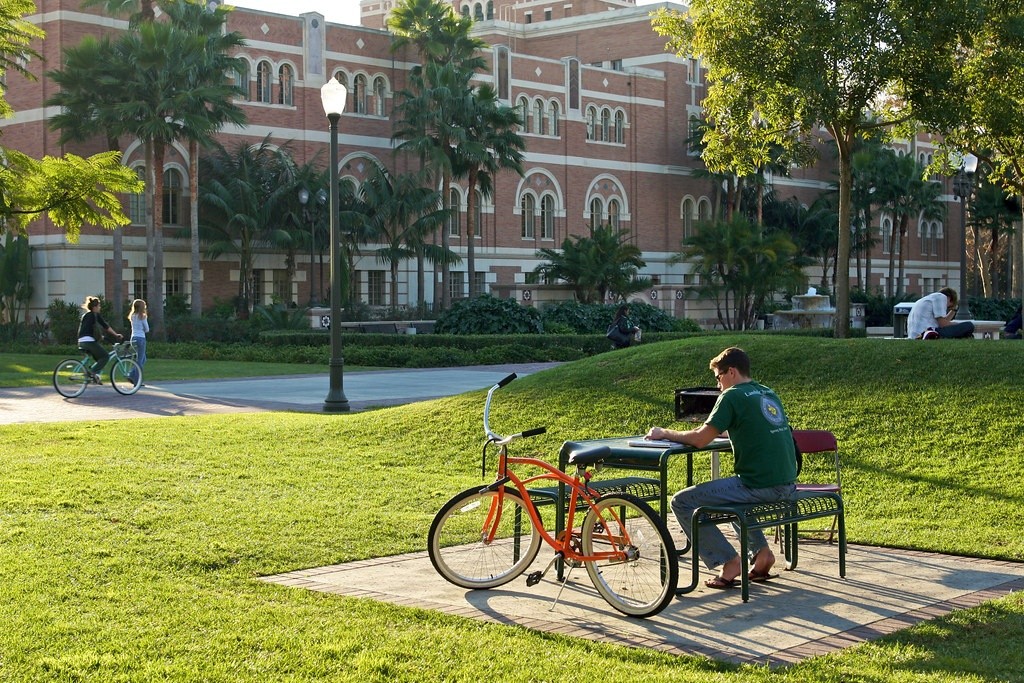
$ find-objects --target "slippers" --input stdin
[747,571,779,581]
[705,576,741,588]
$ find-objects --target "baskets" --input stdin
[115,340,138,357]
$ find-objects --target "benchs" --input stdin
[359,323,398,333]
[673,491,847,604]
[410,323,435,334]
[513,477,684,570]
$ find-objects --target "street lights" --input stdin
[951,151,978,320]
[319,75,353,414]
[299,186,327,308]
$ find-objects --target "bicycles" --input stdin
[52,333,144,398]
[426,373,678,621]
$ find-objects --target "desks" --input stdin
[556,435,791,587]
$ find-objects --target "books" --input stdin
[631,439,671,448]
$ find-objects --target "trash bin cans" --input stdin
[892,302,913,338]
[849,303,866,330]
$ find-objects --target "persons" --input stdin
[611,304,640,349]
[127,299,150,387]
[907,289,974,341]
[77,296,124,385]
[644,347,796,589]
[1004,305,1024,339]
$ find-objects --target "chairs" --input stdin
[774,430,848,554]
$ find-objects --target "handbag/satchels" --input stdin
[606,325,631,345]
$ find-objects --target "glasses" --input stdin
[715,365,735,382]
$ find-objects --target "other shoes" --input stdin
[134,384,145,387]
[86,371,102,385]
[128,376,134,384]
